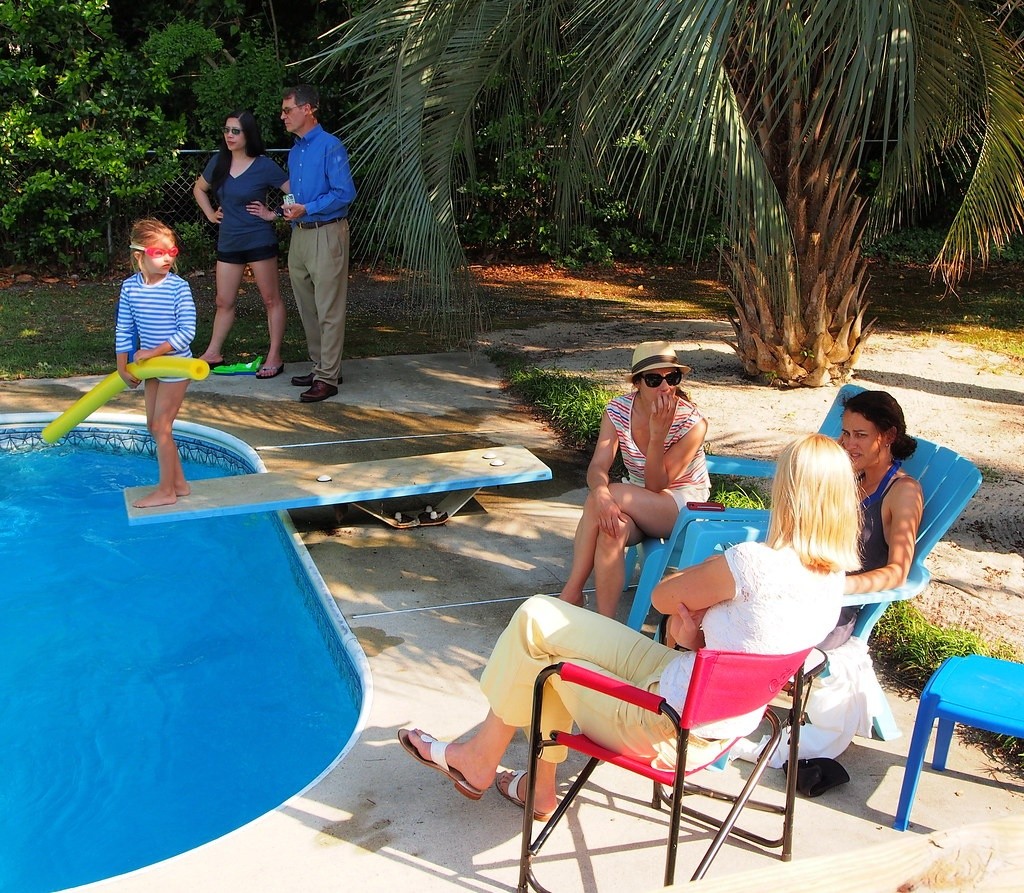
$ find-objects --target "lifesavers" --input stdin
[40,355,210,445]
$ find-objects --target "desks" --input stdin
[662,614,830,695]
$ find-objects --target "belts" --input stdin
[295,218,342,230]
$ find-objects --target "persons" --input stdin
[811,390,927,652]
[394,431,861,822]
[557,341,713,618]
[115,219,197,507]
[191,109,291,378]
[278,86,357,405]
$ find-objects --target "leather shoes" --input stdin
[299,380,338,402]
[291,371,343,386]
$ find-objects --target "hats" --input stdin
[625,340,691,383]
[783,757,850,798]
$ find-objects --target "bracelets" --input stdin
[273,206,285,218]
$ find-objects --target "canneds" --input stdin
[283,193,295,213]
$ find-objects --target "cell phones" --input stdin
[687,502,725,511]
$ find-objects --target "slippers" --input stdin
[397,728,484,800]
[209,361,225,370]
[497,770,551,823]
[255,363,285,379]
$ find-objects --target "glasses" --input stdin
[280,103,306,115]
[128,244,179,258]
[641,370,683,388]
[220,126,245,135]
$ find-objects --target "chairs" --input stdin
[658,436,984,772]
[518,639,817,893]
[616,384,879,635]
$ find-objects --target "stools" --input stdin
[894,654,1024,834]
